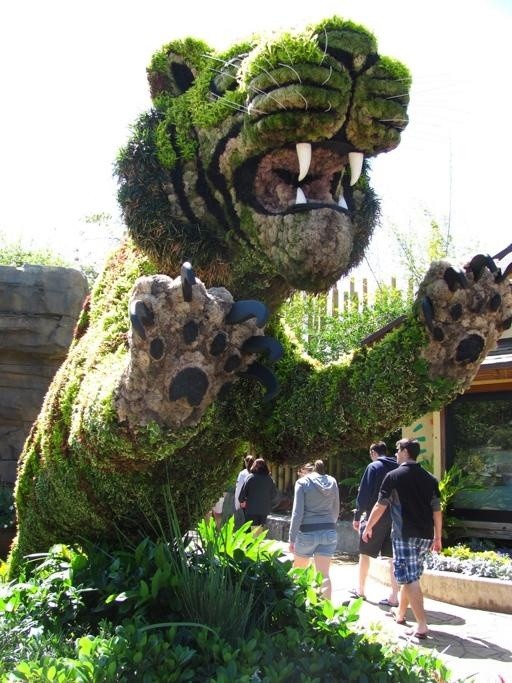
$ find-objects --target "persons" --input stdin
[237,457,281,547]
[287,458,342,607]
[348,437,403,607]
[204,489,224,534]
[233,453,257,534]
[359,436,445,638]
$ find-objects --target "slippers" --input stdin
[379,598,405,623]
[403,627,427,639]
[347,588,366,600]
